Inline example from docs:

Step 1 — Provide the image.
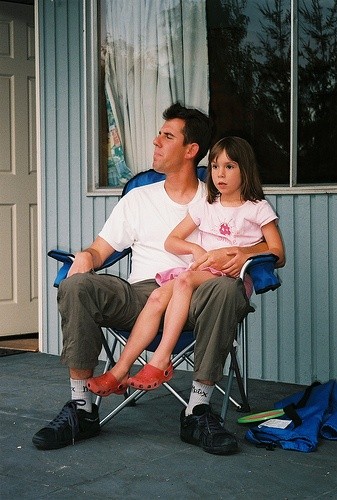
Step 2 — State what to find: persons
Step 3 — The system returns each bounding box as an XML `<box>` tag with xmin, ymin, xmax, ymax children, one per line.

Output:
<box><xmin>86</xmin><ymin>137</ymin><xmax>285</xmax><ymax>396</ymax></box>
<box><xmin>31</xmin><ymin>99</ymin><xmax>268</xmax><ymax>456</ymax></box>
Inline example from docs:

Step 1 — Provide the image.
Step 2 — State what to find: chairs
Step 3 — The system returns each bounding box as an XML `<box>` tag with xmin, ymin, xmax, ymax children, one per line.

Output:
<box><xmin>46</xmin><ymin>167</ymin><xmax>283</xmax><ymax>429</ymax></box>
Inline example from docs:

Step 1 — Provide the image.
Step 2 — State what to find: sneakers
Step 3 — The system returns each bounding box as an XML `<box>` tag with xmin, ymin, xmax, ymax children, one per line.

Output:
<box><xmin>180</xmin><ymin>403</ymin><xmax>238</xmax><ymax>453</ymax></box>
<box><xmin>31</xmin><ymin>399</ymin><xmax>101</xmax><ymax>449</ymax></box>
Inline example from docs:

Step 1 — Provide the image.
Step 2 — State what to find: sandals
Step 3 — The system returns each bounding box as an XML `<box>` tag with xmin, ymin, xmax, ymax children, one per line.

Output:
<box><xmin>87</xmin><ymin>370</ymin><xmax>126</xmax><ymax>397</ymax></box>
<box><xmin>127</xmin><ymin>361</ymin><xmax>175</xmax><ymax>390</ymax></box>
